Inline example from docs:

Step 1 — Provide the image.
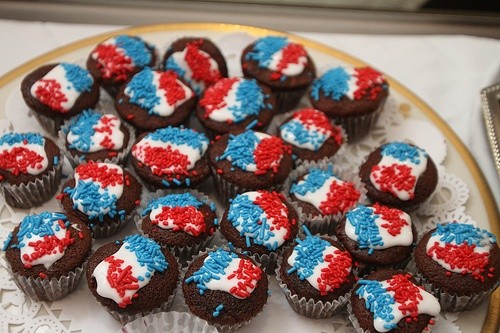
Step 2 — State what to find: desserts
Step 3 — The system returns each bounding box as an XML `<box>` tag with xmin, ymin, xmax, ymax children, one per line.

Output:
<box><xmin>0</xmin><ymin>34</ymin><xmax>500</xmax><ymax>333</ymax></box>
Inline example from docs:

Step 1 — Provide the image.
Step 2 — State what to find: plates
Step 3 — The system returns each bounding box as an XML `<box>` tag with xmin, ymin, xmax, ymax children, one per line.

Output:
<box><xmin>0</xmin><ymin>23</ymin><xmax>500</xmax><ymax>333</ymax></box>
